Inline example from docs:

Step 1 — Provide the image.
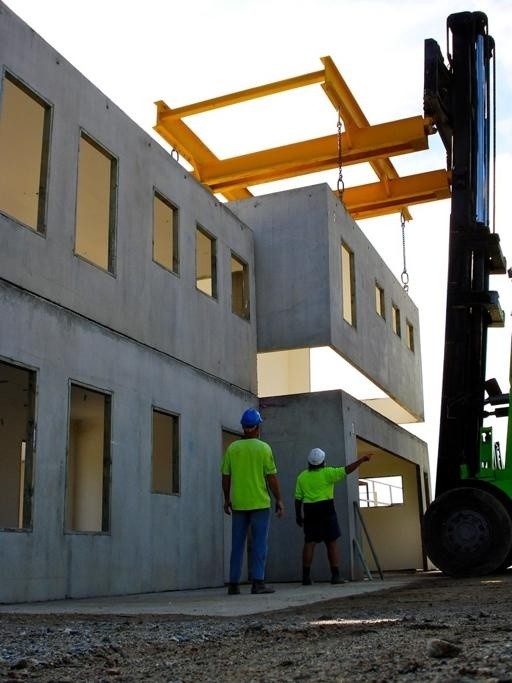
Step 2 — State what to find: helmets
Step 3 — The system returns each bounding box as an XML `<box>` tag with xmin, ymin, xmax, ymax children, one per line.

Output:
<box><xmin>306</xmin><ymin>447</ymin><xmax>326</xmax><ymax>466</ymax></box>
<box><xmin>240</xmin><ymin>407</ymin><xmax>263</xmax><ymax>425</ymax></box>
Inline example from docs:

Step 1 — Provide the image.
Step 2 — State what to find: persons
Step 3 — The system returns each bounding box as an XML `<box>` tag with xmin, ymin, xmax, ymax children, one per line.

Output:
<box><xmin>222</xmin><ymin>408</ymin><xmax>284</xmax><ymax>594</ymax></box>
<box><xmin>294</xmin><ymin>448</ymin><xmax>372</xmax><ymax>585</ymax></box>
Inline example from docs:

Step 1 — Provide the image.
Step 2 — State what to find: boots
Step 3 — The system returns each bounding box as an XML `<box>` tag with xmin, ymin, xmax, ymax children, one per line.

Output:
<box><xmin>302</xmin><ymin>565</ymin><xmax>312</xmax><ymax>585</ymax></box>
<box><xmin>331</xmin><ymin>566</ymin><xmax>350</xmax><ymax>583</ymax></box>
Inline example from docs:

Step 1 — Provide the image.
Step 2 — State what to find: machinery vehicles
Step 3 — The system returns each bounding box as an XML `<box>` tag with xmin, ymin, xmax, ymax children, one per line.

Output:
<box><xmin>152</xmin><ymin>10</ymin><xmax>511</xmax><ymax>586</ymax></box>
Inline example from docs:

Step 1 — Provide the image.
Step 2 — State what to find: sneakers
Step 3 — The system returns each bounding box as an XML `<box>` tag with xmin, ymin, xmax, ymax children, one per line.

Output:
<box><xmin>251</xmin><ymin>582</ymin><xmax>275</xmax><ymax>594</ymax></box>
<box><xmin>228</xmin><ymin>583</ymin><xmax>241</xmax><ymax>595</ymax></box>
